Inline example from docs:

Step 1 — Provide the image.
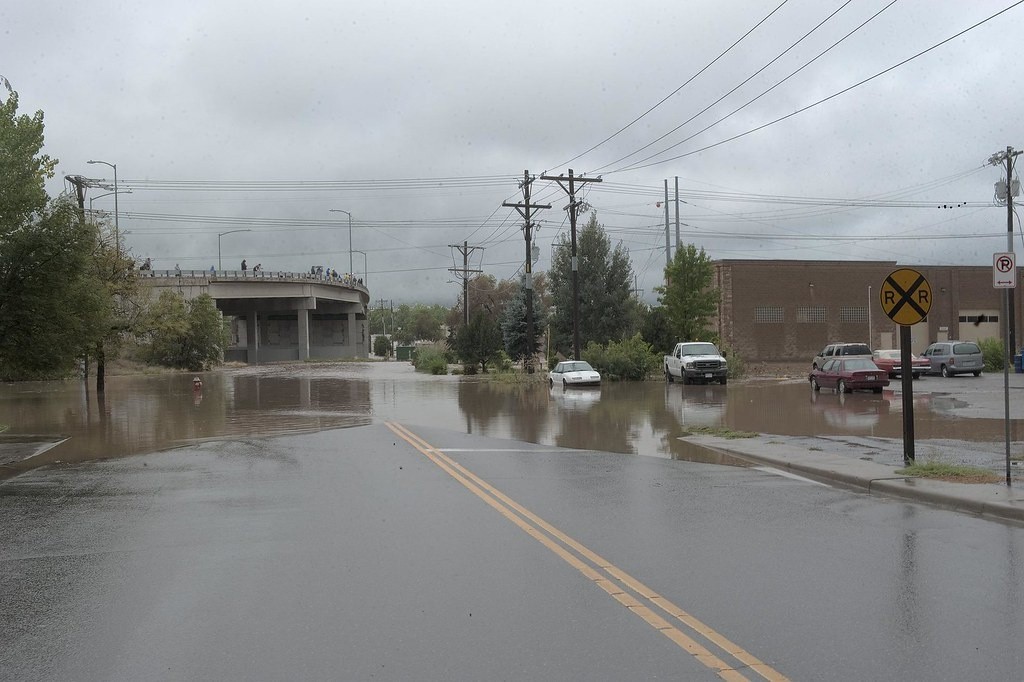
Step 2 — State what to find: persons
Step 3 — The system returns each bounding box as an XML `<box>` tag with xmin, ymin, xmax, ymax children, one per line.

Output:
<box><xmin>140</xmin><ymin>257</ymin><xmax>151</xmax><ymax>270</ymax></box>
<box><xmin>241</xmin><ymin>259</ymin><xmax>247</xmax><ymax>276</ymax></box>
<box><xmin>174</xmin><ymin>264</ymin><xmax>180</xmax><ymax>277</ymax></box>
<box><xmin>210</xmin><ymin>266</ymin><xmax>216</xmax><ymax>278</ymax></box>
<box><xmin>311</xmin><ymin>266</ymin><xmax>363</xmax><ymax>285</ymax></box>
<box><xmin>253</xmin><ymin>264</ymin><xmax>261</xmax><ymax>276</ymax></box>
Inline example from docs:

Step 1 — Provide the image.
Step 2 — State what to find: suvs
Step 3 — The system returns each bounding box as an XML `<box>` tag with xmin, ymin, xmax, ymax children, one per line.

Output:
<box><xmin>817</xmin><ymin>343</ymin><xmax>873</xmax><ymax>360</ymax></box>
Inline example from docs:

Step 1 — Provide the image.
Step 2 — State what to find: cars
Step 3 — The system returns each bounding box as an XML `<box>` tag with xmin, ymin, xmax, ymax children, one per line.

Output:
<box><xmin>547</xmin><ymin>360</ymin><xmax>601</xmax><ymax>387</ymax></box>
<box><xmin>808</xmin><ymin>356</ymin><xmax>890</xmax><ymax>394</ymax></box>
<box><xmin>872</xmin><ymin>349</ymin><xmax>932</xmax><ymax>379</ymax></box>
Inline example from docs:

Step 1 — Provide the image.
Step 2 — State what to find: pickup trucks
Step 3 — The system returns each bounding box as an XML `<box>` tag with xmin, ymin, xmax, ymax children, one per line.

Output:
<box><xmin>663</xmin><ymin>342</ymin><xmax>729</xmax><ymax>385</ymax></box>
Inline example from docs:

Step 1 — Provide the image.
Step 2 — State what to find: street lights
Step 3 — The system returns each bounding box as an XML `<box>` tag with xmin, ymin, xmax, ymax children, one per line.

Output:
<box><xmin>87</xmin><ymin>160</ymin><xmax>119</xmax><ymax>258</ymax></box>
<box><xmin>329</xmin><ymin>209</ymin><xmax>352</xmax><ymax>282</ymax></box>
<box><xmin>89</xmin><ymin>191</ymin><xmax>136</xmax><ymax>226</ymax></box>
<box><xmin>351</xmin><ymin>250</ymin><xmax>367</xmax><ymax>288</ymax></box>
<box><xmin>219</xmin><ymin>229</ymin><xmax>251</xmax><ymax>277</ymax></box>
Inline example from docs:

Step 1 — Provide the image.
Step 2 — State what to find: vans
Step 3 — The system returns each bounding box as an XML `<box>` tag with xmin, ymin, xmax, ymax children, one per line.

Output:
<box><xmin>920</xmin><ymin>341</ymin><xmax>985</xmax><ymax>378</ymax></box>
